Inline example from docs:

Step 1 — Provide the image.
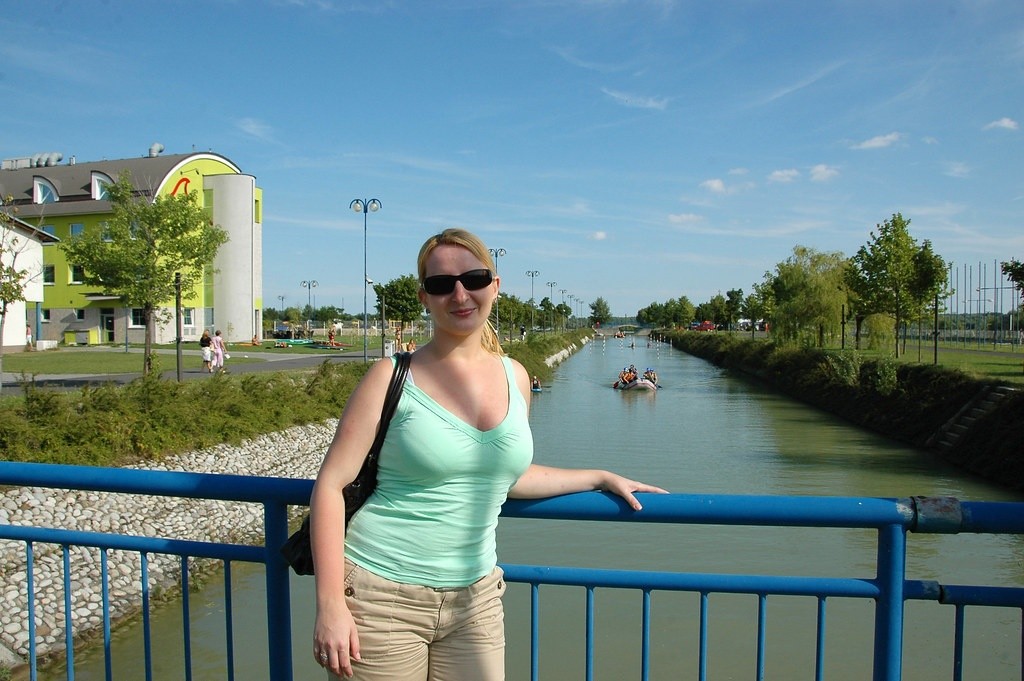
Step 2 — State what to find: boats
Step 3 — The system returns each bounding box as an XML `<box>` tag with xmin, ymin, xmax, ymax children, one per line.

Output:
<box><xmin>622</xmin><ymin>376</ymin><xmax>657</xmax><ymax>391</ymax></box>
<box><xmin>593</xmin><ymin>333</ymin><xmax>604</xmax><ymax>337</ymax></box>
<box><xmin>531</xmin><ymin>386</ymin><xmax>542</xmax><ymax>393</ymax></box>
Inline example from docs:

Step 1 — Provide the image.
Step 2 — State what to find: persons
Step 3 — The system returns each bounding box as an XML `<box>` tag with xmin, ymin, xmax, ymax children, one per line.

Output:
<box><xmin>198</xmin><ymin>330</ymin><xmax>213</xmax><ymax>373</ymax></box>
<box><xmin>310</xmin><ymin>228</ymin><xmax>671</xmax><ymax>681</ymax></box>
<box><xmin>26</xmin><ymin>324</ymin><xmax>33</xmax><ymax>352</ymax></box>
<box><xmin>209</xmin><ymin>330</ymin><xmax>228</xmax><ymax>374</ymax></box>
<box><xmin>252</xmin><ymin>335</ymin><xmax>262</xmax><ymax>346</ymax></box>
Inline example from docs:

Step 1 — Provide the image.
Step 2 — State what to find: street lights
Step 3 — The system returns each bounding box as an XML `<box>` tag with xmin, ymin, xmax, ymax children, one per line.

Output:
<box><xmin>300</xmin><ymin>279</ymin><xmax>319</xmax><ymax>320</ymax></box>
<box><xmin>525</xmin><ymin>270</ymin><xmax>540</xmax><ymax>328</ymax></box>
<box><xmin>545</xmin><ymin>281</ymin><xmax>557</xmax><ymax>332</ymax></box>
<box><xmin>278</xmin><ymin>295</ymin><xmax>286</xmax><ymax>312</ymax></box>
<box><xmin>568</xmin><ymin>295</ymin><xmax>585</xmax><ymax>329</ymax></box>
<box><xmin>962</xmin><ymin>298</ymin><xmax>993</xmax><ymax>343</ymax></box>
<box><xmin>348</xmin><ymin>197</ymin><xmax>382</xmax><ymax>363</ymax></box>
<box><xmin>487</xmin><ymin>248</ymin><xmax>507</xmax><ymax>329</ymax></box>
<box><xmin>559</xmin><ymin>289</ymin><xmax>567</xmax><ymax>333</ymax></box>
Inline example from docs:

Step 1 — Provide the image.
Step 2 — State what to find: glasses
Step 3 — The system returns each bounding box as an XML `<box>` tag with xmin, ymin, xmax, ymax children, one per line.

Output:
<box><xmin>424</xmin><ymin>268</ymin><xmax>494</xmax><ymax>295</ymax></box>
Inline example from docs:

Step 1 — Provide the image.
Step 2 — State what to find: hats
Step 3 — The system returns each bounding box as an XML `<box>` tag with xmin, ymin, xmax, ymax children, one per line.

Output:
<box><xmin>650</xmin><ymin>369</ymin><xmax>653</xmax><ymax>372</ymax></box>
<box><xmin>624</xmin><ymin>367</ymin><xmax>628</xmax><ymax>371</ymax></box>
<box><xmin>647</xmin><ymin>368</ymin><xmax>650</xmax><ymax>371</ymax></box>
<box><xmin>630</xmin><ymin>364</ymin><xmax>634</xmax><ymax>368</ymax></box>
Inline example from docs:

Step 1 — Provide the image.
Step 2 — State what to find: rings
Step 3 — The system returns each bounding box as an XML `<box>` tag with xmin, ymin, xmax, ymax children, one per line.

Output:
<box><xmin>319</xmin><ymin>653</ymin><xmax>328</xmax><ymax>659</ymax></box>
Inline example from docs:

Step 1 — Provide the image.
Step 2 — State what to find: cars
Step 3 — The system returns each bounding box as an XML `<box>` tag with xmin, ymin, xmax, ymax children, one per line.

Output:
<box><xmin>690</xmin><ymin>320</ymin><xmax>767</xmax><ymax>331</ymax></box>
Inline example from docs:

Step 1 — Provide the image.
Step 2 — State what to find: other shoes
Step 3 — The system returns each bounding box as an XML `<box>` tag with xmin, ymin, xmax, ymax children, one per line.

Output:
<box><xmin>209</xmin><ymin>367</ymin><xmax>212</xmax><ymax>373</ymax></box>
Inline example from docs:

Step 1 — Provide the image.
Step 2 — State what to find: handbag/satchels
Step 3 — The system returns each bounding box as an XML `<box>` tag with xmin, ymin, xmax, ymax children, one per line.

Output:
<box><xmin>282</xmin><ymin>350</ymin><xmax>412</xmax><ymax>575</ymax></box>
